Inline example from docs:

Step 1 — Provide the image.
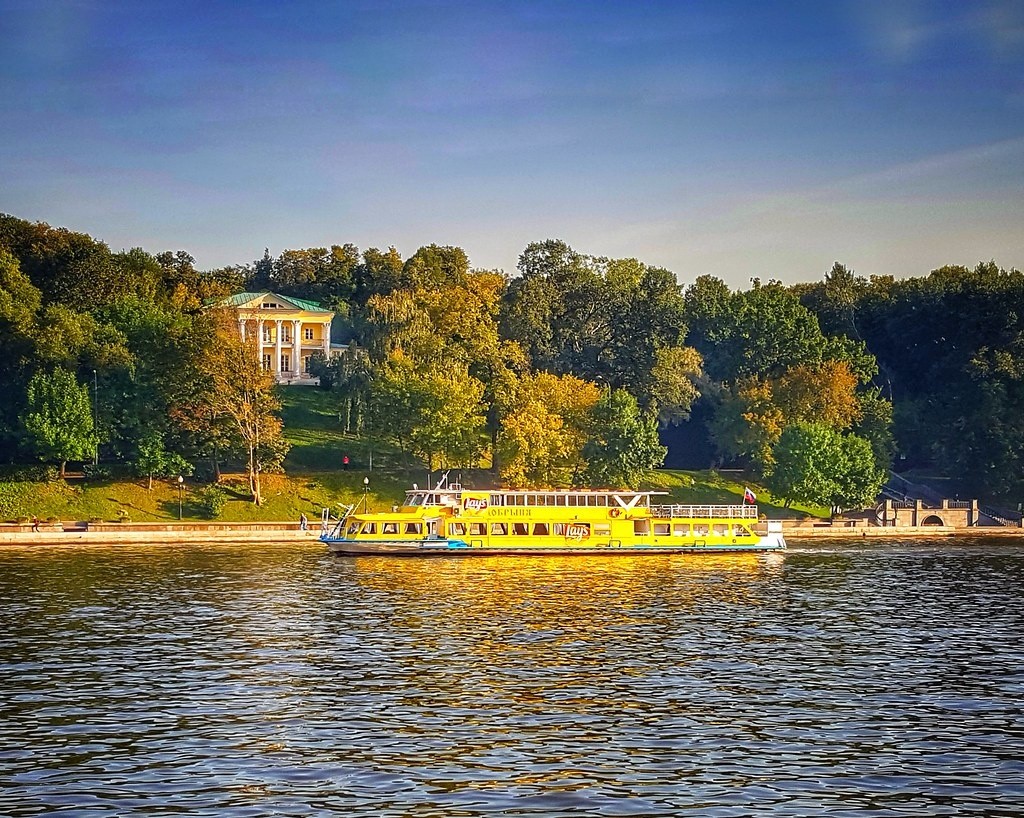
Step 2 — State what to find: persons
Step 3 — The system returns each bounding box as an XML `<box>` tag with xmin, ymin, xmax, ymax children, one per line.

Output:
<box><xmin>343</xmin><ymin>455</ymin><xmax>349</xmax><ymax>470</ymax></box>
<box><xmin>691</xmin><ymin>478</ymin><xmax>694</xmax><ymax>487</ymax></box>
<box><xmin>300</xmin><ymin>513</ymin><xmax>308</xmax><ymax>530</ymax></box>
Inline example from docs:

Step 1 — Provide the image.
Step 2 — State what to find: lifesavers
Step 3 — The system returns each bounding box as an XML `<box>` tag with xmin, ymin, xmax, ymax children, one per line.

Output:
<box><xmin>611</xmin><ymin>508</ymin><xmax>620</xmax><ymax>518</ymax></box>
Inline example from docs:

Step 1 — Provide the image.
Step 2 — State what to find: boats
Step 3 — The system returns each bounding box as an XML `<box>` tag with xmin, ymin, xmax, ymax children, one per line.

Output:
<box><xmin>320</xmin><ymin>474</ymin><xmax>786</xmax><ymax>557</ymax></box>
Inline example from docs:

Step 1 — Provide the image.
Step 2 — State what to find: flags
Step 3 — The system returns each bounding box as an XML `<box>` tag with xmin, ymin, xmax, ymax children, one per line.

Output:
<box><xmin>745</xmin><ymin>488</ymin><xmax>756</xmax><ymax>504</ymax></box>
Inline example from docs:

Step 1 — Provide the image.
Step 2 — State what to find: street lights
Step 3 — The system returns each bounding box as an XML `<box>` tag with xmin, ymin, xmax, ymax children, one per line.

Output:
<box><xmin>363</xmin><ymin>476</ymin><xmax>369</xmax><ymax>514</ymax></box>
<box><xmin>691</xmin><ymin>478</ymin><xmax>694</xmax><ymax>502</ymax></box>
<box><xmin>90</xmin><ymin>369</ymin><xmax>102</xmax><ymax>465</ymax></box>
<box><xmin>175</xmin><ymin>475</ymin><xmax>187</xmax><ymax>520</ymax></box>
